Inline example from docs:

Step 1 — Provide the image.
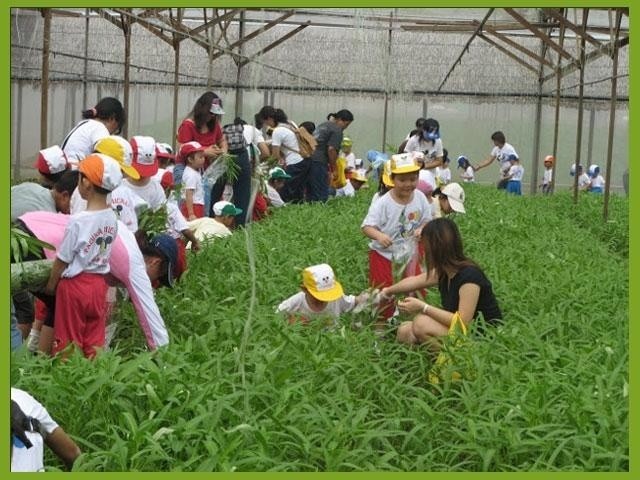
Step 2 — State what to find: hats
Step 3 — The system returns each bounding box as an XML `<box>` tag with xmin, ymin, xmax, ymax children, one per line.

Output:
<box><xmin>213</xmin><ymin>200</ymin><xmax>244</xmax><ymax>216</ymax></box>
<box><xmin>351</xmin><ymin>150</ymin><xmax>388</xmax><ymax>183</ymax></box>
<box><xmin>302</xmin><ymin>264</ymin><xmax>343</xmax><ymax>302</ymax></box>
<box><xmin>268</xmin><ymin>167</ymin><xmax>291</xmax><ymax>179</ymax></box>
<box><xmin>441</xmin><ymin>183</ymin><xmax>466</xmax><ymax>214</ymax></box>
<box><xmin>543</xmin><ymin>155</ymin><xmax>553</xmax><ymax>162</ymax></box>
<box><xmin>457</xmin><ymin>156</ymin><xmax>468</xmax><ymax>169</ymax></box>
<box><xmin>150</xmin><ymin>233</ymin><xmax>176</xmax><ymax>289</ymax></box>
<box><xmin>31</xmin><ymin>145</ymin><xmax>68</xmax><ymax>174</ymax></box>
<box><xmin>423</xmin><ymin>119</ymin><xmax>439</xmax><ymax>140</ymax></box>
<box><xmin>382</xmin><ymin>151</ymin><xmax>426</xmax><ymax>187</ymax></box>
<box><xmin>208</xmin><ymin>98</ymin><xmax>225</xmax><ymax>115</ymax></box>
<box><xmin>569</xmin><ymin>164</ymin><xmax>599</xmax><ymax>176</ymax></box>
<box><xmin>505</xmin><ymin>154</ymin><xmax>520</xmax><ymax>160</ymax></box>
<box><xmin>66</xmin><ymin>135</ymin><xmax>209</xmax><ymax>188</ymax></box>
<box><xmin>340</xmin><ymin>137</ymin><xmax>352</xmax><ymax>148</ymax></box>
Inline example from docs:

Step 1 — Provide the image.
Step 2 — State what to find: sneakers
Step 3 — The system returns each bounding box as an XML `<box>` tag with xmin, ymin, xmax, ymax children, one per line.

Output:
<box><xmin>26</xmin><ymin>329</ymin><xmax>40</xmax><ymax>352</ymax></box>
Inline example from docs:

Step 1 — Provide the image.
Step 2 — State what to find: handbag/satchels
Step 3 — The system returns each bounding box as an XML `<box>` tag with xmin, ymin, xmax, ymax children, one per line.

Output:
<box><xmin>428</xmin><ymin>312</ymin><xmax>468</xmax><ymax>389</ymax></box>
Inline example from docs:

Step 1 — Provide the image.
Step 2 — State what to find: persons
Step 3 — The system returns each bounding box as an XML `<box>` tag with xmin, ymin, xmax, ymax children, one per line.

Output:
<box><xmin>569</xmin><ymin>163</ymin><xmax>591</xmax><ymax>193</ymax></box>
<box><xmin>472</xmin><ymin>131</ymin><xmax>520</xmax><ymax>190</ymax></box>
<box><xmin>587</xmin><ymin>164</ymin><xmax>606</xmax><ymax>194</ymax></box>
<box><xmin>374</xmin><ymin>215</ymin><xmax>508</xmax><ymax>354</ymax></box>
<box><xmin>361</xmin><ymin>117</ymin><xmax>468</xmax><ymax>320</ymax></box>
<box><xmin>9</xmin><ymin>89</ymin><xmax>370</xmax><ymax>361</ymax></box>
<box><xmin>538</xmin><ymin>155</ymin><xmax>556</xmax><ymax>195</ymax></box>
<box><xmin>500</xmin><ymin>153</ymin><xmax>526</xmax><ymax>196</ymax></box>
<box><xmin>456</xmin><ymin>155</ymin><xmax>475</xmax><ymax>184</ymax></box>
<box><xmin>10</xmin><ymin>385</ymin><xmax>84</xmax><ymax>473</ymax></box>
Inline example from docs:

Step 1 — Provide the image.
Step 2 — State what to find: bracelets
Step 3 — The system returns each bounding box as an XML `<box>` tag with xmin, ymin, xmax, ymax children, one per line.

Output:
<box><xmin>422</xmin><ymin>303</ymin><xmax>430</xmax><ymax>314</ymax></box>
<box><xmin>381</xmin><ymin>287</ymin><xmax>395</xmax><ymax>300</ymax></box>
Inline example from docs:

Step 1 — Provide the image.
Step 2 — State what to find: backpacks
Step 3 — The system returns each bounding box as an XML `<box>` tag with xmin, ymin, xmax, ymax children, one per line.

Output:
<box><xmin>223</xmin><ymin>124</ymin><xmax>246</xmax><ymax>154</ymax></box>
<box><xmin>295</xmin><ymin>127</ymin><xmax>317</xmax><ymax>158</ymax></box>
